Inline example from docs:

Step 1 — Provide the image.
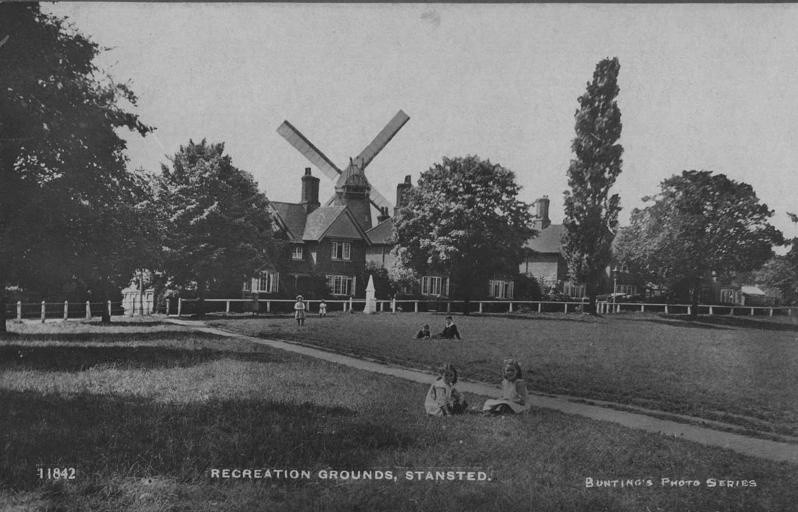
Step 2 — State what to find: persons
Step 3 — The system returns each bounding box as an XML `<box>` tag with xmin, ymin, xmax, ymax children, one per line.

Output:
<box><xmin>250</xmin><ymin>292</ymin><xmax>260</xmax><ymax>316</ymax></box>
<box><xmin>414</xmin><ymin>323</ymin><xmax>429</xmax><ymax>340</ymax></box>
<box><xmin>482</xmin><ymin>358</ymin><xmax>530</xmax><ymax>416</ymax></box>
<box><xmin>430</xmin><ymin>315</ymin><xmax>463</xmax><ymax>340</ymax></box>
<box><xmin>319</xmin><ymin>298</ymin><xmax>327</xmax><ymax>317</ymax></box>
<box><xmin>423</xmin><ymin>363</ymin><xmax>468</xmax><ymax>417</ymax></box>
<box><xmin>293</xmin><ymin>294</ymin><xmax>306</xmax><ymax>326</ymax></box>
